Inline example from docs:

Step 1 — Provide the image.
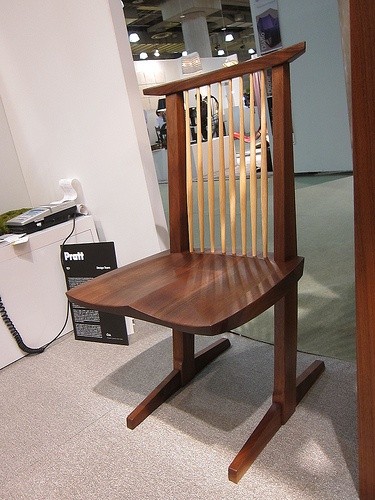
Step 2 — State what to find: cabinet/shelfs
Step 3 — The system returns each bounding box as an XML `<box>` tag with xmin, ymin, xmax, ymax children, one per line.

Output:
<box><xmin>0</xmin><ymin>215</ymin><xmax>101</xmax><ymax>369</ymax></box>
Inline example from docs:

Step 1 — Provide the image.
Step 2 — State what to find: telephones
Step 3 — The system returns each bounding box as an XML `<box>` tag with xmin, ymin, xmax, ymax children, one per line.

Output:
<box><xmin>5</xmin><ymin>200</ymin><xmax>77</xmax><ymax>234</ymax></box>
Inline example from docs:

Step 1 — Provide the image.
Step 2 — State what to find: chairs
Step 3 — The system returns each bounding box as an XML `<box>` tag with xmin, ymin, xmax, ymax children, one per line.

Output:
<box><xmin>65</xmin><ymin>41</ymin><xmax>326</xmax><ymax>484</ymax></box>
